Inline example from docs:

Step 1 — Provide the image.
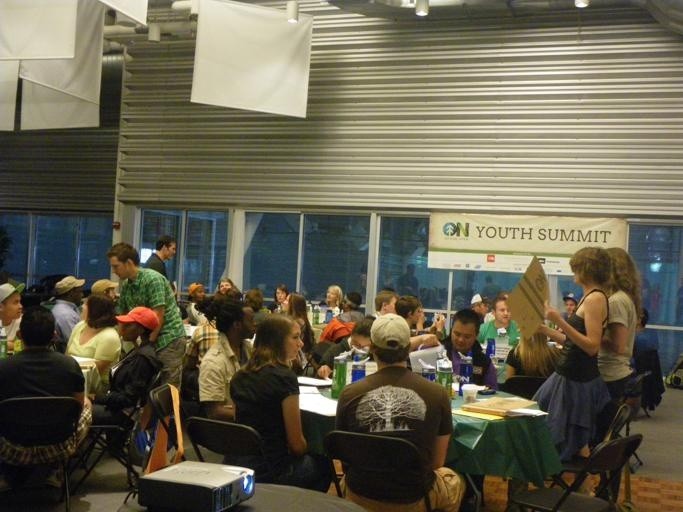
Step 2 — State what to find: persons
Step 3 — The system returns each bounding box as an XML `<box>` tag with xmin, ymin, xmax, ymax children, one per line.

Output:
<box><xmin>3</xmin><ymin>276</ymin><xmax>162</xmax><ymax>512</ymax></box>
<box><xmin>335</xmin><ymin>313</ymin><xmax>466</xmax><ymax>510</ymax></box>
<box><xmin>478</xmin><ymin>290</ymin><xmax>518</xmax><ymax>347</ymax></box>
<box><xmin>587</xmin><ymin>246</ymin><xmax>645</xmax><ymax>496</ymax></box>
<box><xmin>142</xmin><ymin>234</ymin><xmax>177</xmax><ymax>280</ymax></box>
<box><xmin>223</xmin><ymin>313</ymin><xmax>333</xmax><ymax>494</ymax></box>
<box><xmin>505</xmin><ymin>321</ymin><xmax>564</xmax><ymax>379</ymax></box>
<box><xmin>531</xmin><ymin>246</ymin><xmax>612</xmax><ymax>497</ymax></box>
<box><xmin>108</xmin><ymin>243</ymin><xmax>187</xmax><ymax>450</ymax></box>
<box><xmin>633</xmin><ymin>307</ymin><xmax>660</xmax><ymax>352</ymax></box>
<box><xmin>560</xmin><ymin>292</ymin><xmax>579</xmax><ymax>321</ymax></box>
<box><xmin>183</xmin><ymin>276</ymin><xmax>497</xmax><ymax>422</ymax></box>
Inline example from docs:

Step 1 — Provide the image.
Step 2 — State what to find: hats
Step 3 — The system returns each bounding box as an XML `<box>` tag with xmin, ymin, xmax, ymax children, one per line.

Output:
<box><xmin>187</xmin><ymin>283</ymin><xmax>203</xmax><ymax>301</ymax></box>
<box><xmin>344</xmin><ymin>292</ymin><xmax>362</xmax><ymax>309</ymax></box>
<box><xmin>371</xmin><ymin>314</ymin><xmax>410</xmax><ymax>349</ymax></box>
<box><xmin>92</xmin><ymin>280</ymin><xmax>118</xmax><ymax>298</ymax></box>
<box><xmin>0</xmin><ymin>283</ymin><xmax>25</xmax><ymax>304</ymax></box>
<box><xmin>116</xmin><ymin>307</ymin><xmax>159</xmax><ymax>331</ymax></box>
<box><xmin>55</xmin><ymin>275</ymin><xmax>86</xmax><ymax>295</ymax></box>
<box><xmin>470</xmin><ymin>293</ymin><xmax>489</xmax><ymax>306</ymax></box>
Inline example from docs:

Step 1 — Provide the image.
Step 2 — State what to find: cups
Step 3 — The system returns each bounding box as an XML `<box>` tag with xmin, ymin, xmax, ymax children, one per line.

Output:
<box><xmin>461</xmin><ymin>384</ymin><xmax>477</xmax><ymax>402</ymax></box>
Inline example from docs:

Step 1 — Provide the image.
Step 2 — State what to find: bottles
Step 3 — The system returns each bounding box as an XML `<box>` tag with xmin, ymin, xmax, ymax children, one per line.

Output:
<box><xmin>436</xmin><ymin>361</ymin><xmax>453</xmax><ymax>399</ymax></box>
<box><xmin>331</xmin><ymin>355</ymin><xmax>347</xmax><ymax>397</ymax></box>
<box><xmin>458</xmin><ymin>352</ymin><xmax>473</xmax><ymax>396</ymax></box>
<box><xmin>486</xmin><ymin>338</ymin><xmax>495</xmax><ymax>359</ymax></box>
<box><xmin>312</xmin><ymin>305</ymin><xmax>319</xmax><ymax>325</ymax></box>
<box><xmin>325</xmin><ymin>310</ymin><xmax>332</xmax><ymax>323</ymax></box>
<box><xmin>0</xmin><ymin>333</ymin><xmax>7</xmax><ymax>361</ymax></box>
<box><xmin>351</xmin><ymin>361</ymin><xmax>365</xmax><ymax>381</ymax></box>
<box><xmin>421</xmin><ymin>367</ymin><xmax>435</xmax><ymax>383</ymax></box>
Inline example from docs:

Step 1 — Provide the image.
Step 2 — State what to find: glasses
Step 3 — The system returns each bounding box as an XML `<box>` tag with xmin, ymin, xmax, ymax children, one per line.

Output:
<box><xmin>562</xmin><ymin>292</ymin><xmax>577</xmax><ymax>305</ymax></box>
<box><xmin>350</xmin><ymin>342</ymin><xmax>370</xmax><ymax>349</ymax></box>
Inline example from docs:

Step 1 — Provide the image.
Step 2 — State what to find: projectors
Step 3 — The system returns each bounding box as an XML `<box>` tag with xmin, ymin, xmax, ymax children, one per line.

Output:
<box><xmin>138</xmin><ymin>461</ymin><xmax>255</xmax><ymax>512</ymax></box>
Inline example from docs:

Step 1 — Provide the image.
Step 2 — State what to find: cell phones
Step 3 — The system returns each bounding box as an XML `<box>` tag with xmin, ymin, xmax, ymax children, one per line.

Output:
<box><xmin>478</xmin><ymin>388</ymin><xmax>496</xmax><ymax>395</ymax></box>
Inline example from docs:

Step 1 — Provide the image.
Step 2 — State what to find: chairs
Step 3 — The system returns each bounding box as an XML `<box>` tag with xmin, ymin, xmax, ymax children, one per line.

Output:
<box><xmin>0</xmin><ymin>324</ymin><xmax>653</xmax><ymax>512</ymax></box>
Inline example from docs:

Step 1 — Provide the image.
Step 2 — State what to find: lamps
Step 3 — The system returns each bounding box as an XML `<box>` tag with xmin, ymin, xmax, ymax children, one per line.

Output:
<box><xmin>146</xmin><ymin>21</ymin><xmax>161</xmax><ymax>44</ymax></box>
<box><xmin>168</xmin><ymin>1</ymin><xmax>592</xmax><ymax>26</ymax></box>
